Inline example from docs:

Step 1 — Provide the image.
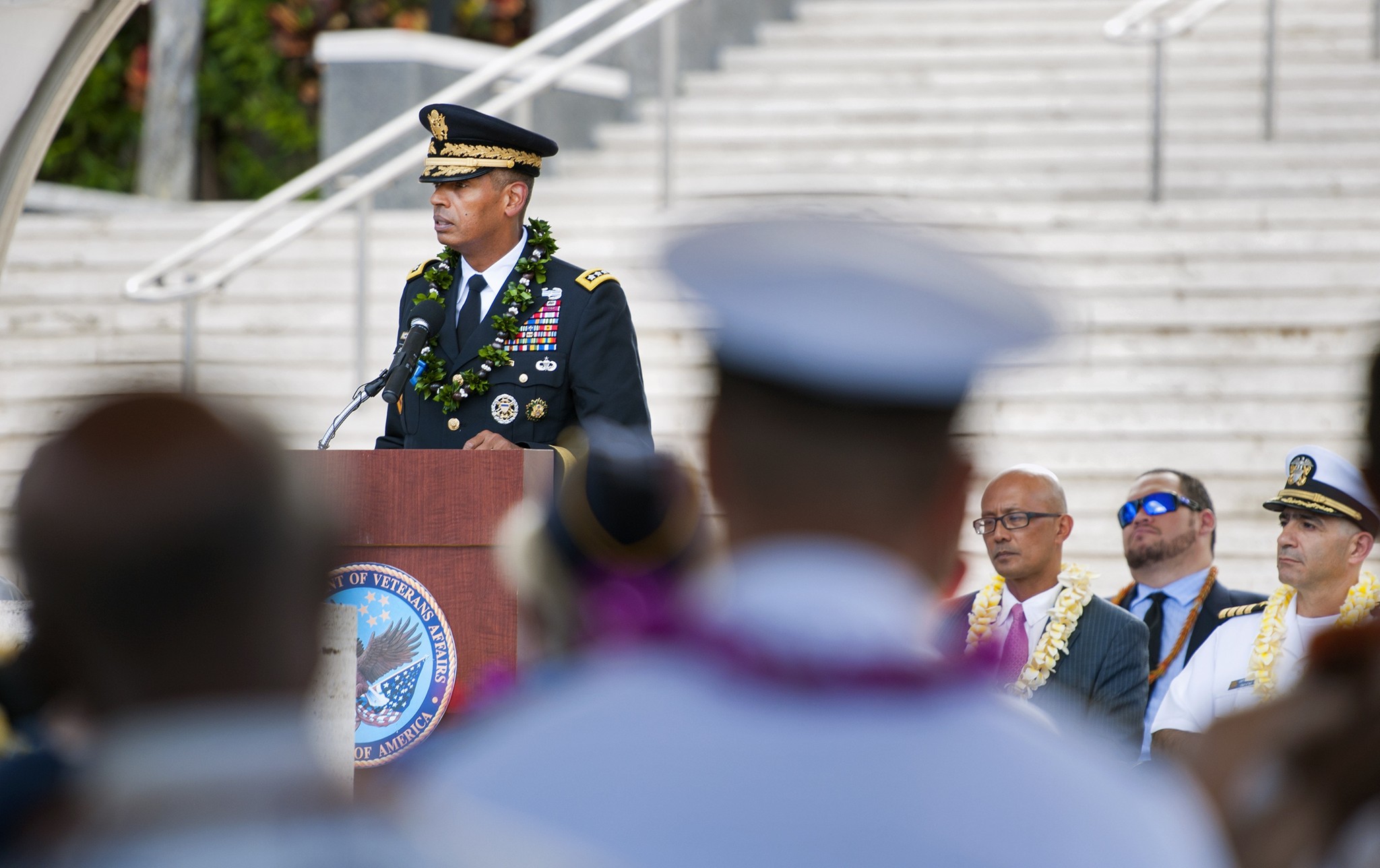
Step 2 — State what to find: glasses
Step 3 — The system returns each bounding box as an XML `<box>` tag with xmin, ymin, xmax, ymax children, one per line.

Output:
<box><xmin>972</xmin><ymin>511</ymin><xmax>1066</xmax><ymax>534</ymax></box>
<box><xmin>1117</xmin><ymin>493</ymin><xmax>1204</xmax><ymax>528</ymax></box>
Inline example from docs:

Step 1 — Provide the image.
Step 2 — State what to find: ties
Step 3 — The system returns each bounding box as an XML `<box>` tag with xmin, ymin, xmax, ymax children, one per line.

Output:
<box><xmin>1142</xmin><ymin>589</ymin><xmax>1169</xmax><ymax>714</ymax></box>
<box><xmin>456</xmin><ymin>273</ymin><xmax>488</xmax><ymax>350</ymax></box>
<box><xmin>999</xmin><ymin>603</ymin><xmax>1029</xmax><ymax>686</ymax></box>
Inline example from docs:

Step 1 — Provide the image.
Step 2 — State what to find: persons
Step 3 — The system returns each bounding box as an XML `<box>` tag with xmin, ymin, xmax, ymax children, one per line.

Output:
<box><xmin>0</xmin><ymin>387</ymin><xmax>404</xmax><ymax>868</ymax></box>
<box><xmin>375</xmin><ymin>103</ymin><xmax>653</xmax><ymax>493</ymax></box>
<box><xmin>1102</xmin><ymin>469</ymin><xmax>1267</xmax><ymax>764</ymax></box>
<box><xmin>1151</xmin><ymin>444</ymin><xmax>1380</xmax><ymax>786</ymax></box>
<box><xmin>947</xmin><ymin>465</ymin><xmax>1148</xmax><ymax>766</ymax></box>
<box><xmin>489</xmin><ymin>449</ymin><xmax>710</xmax><ymax>643</ymax></box>
<box><xmin>403</xmin><ymin>218</ymin><xmax>1230</xmax><ymax>867</ymax></box>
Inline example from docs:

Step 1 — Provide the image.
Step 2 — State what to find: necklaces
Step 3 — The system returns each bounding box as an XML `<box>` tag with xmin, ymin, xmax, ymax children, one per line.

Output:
<box><xmin>966</xmin><ymin>562</ymin><xmax>1092</xmax><ymax>701</ymax></box>
<box><xmin>573</xmin><ymin>575</ymin><xmax>1026</xmax><ymax>705</ymax></box>
<box><xmin>412</xmin><ymin>218</ymin><xmax>557</xmax><ymax>414</ymax></box>
<box><xmin>1249</xmin><ymin>571</ymin><xmax>1380</xmax><ymax>704</ymax></box>
<box><xmin>1112</xmin><ymin>566</ymin><xmax>1217</xmax><ymax>681</ymax></box>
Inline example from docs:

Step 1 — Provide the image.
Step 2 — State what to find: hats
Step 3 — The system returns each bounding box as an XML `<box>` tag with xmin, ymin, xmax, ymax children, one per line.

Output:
<box><xmin>1262</xmin><ymin>446</ymin><xmax>1380</xmax><ymax>538</ymax></box>
<box><xmin>490</xmin><ymin>417</ymin><xmax>725</xmax><ymax>638</ymax></box>
<box><xmin>669</xmin><ymin>225</ymin><xmax>1051</xmax><ymax>422</ymax></box>
<box><xmin>417</xmin><ymin>103</ymin><xmax>560</xmax><ymax>181</ymax></box>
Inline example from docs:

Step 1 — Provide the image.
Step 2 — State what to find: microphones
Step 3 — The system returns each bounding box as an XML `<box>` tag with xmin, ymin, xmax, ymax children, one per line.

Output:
<box><xmin>383</xmin><ymin>300</ymin><xmax>442</xmax><ymax>404</ymax></box>
